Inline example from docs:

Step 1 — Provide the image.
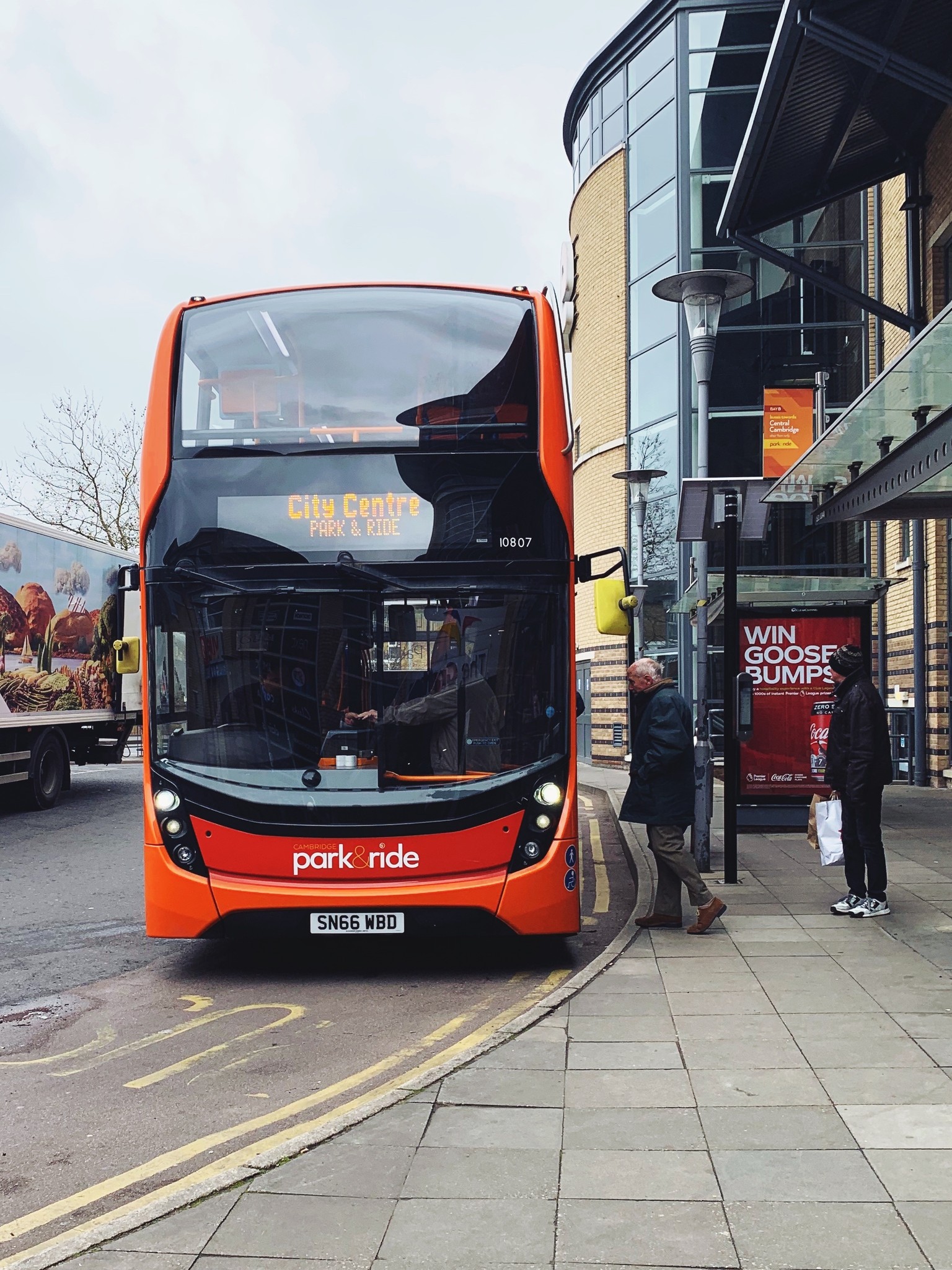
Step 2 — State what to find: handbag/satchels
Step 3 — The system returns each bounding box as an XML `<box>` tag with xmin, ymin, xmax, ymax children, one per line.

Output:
<box><xmin>807</xmin><ymin>791</ymin><xmax>836</xmax><ymax>849</ymax></box>
<box><xmin>814</xmin><ymin>790</ymin><xmax>845</xmax><ymax>866</ymax></box>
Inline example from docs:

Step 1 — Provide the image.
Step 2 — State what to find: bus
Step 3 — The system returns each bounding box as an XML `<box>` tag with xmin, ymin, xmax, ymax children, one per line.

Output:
<box><xmin>113</xmin><ymin>279</ymin><xmax>639</xmax><ymax>939</ymax></box>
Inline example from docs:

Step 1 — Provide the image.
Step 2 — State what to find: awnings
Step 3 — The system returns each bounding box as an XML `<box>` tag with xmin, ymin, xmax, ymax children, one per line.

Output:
<box><xmin>714</xmin><ymin>0</ymin><xmax>952</xmax><ymax>332</ymax></box>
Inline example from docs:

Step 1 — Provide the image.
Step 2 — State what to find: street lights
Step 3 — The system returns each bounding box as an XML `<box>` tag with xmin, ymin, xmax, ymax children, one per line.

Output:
<box><xmin>613</xmin><ymin>468</ymin><xmax>668</xmax><ymax>664</ymax></box>
<box><xmin>652</xmin><ymin>268</ymin><xmax>760</xmax><ymax>874</ymax></box>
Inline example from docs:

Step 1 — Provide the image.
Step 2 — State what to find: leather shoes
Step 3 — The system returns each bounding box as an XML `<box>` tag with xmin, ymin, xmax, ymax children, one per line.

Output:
<box><xmin>634</xmin><ymin>914</ymin><xmax>682</xmax><ymax>928</ymax></box>
<box><xmin>687</xmin><ymin>897</ymin><xmax>727</xmax><ymax>935</ymax></box>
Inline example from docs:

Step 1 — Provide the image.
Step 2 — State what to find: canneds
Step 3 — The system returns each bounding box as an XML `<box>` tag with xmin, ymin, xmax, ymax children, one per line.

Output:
<box><xmin>810</xmin><ymin>701</ymin><xmax>835</xmax><ymax>781</ymax></box>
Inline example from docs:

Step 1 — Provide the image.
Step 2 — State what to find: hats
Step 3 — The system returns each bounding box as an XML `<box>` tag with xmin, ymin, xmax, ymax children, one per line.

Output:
<box><xmin>829</xmin><ymin>643</ymin><xmax>862</xmax><ymax>676</ymax></box>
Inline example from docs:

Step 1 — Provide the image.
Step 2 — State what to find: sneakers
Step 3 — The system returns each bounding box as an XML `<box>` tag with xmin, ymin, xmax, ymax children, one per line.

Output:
<box><xmin>830</xmin><ymin>894</ymin><xmax>866</xmax><ymax>914</ymax></box>
<box><xmin>849</xmin><ymin>899</ymin><xmax>891</xmax><ymax>918</ymax></box>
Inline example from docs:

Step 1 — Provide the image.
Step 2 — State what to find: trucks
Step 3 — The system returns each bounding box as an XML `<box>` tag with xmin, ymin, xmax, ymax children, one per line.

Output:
<box><xmin>0</xmin><ymin>512</ymin><xmax>144</xmax><ymax>811</ymax></box>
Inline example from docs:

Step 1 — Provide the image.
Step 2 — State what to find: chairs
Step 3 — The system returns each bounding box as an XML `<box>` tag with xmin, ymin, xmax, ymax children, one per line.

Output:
<box><xmin>424</xmin><ymin>402</ymin><xmax>530</xmax><ymax>442</ymax></box>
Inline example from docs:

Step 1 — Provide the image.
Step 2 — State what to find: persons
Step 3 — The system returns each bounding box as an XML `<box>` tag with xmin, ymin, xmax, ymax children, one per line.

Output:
<box><xmin>218</xmin><ymin>654</ymin><xmax>357</xmax><ymax>768</ymax></box>
<box><xmin>357</xmin><ymin>647</ymin><xmax>503</xmax><ymax>785</ymax></box>
<box><xmin>576</xmin><ymin>691</ymin><xmax>585</xmax><ymax>718</ymax></box>
<box><xmin>824</xmin><ymin>643</ymin><xmax>891</xmax><ymax>917</ymax></box>
<box><xmin>616</xmin><ymin>658</ymin><xmax>728</xmax><ymax>934</ymax></box>
